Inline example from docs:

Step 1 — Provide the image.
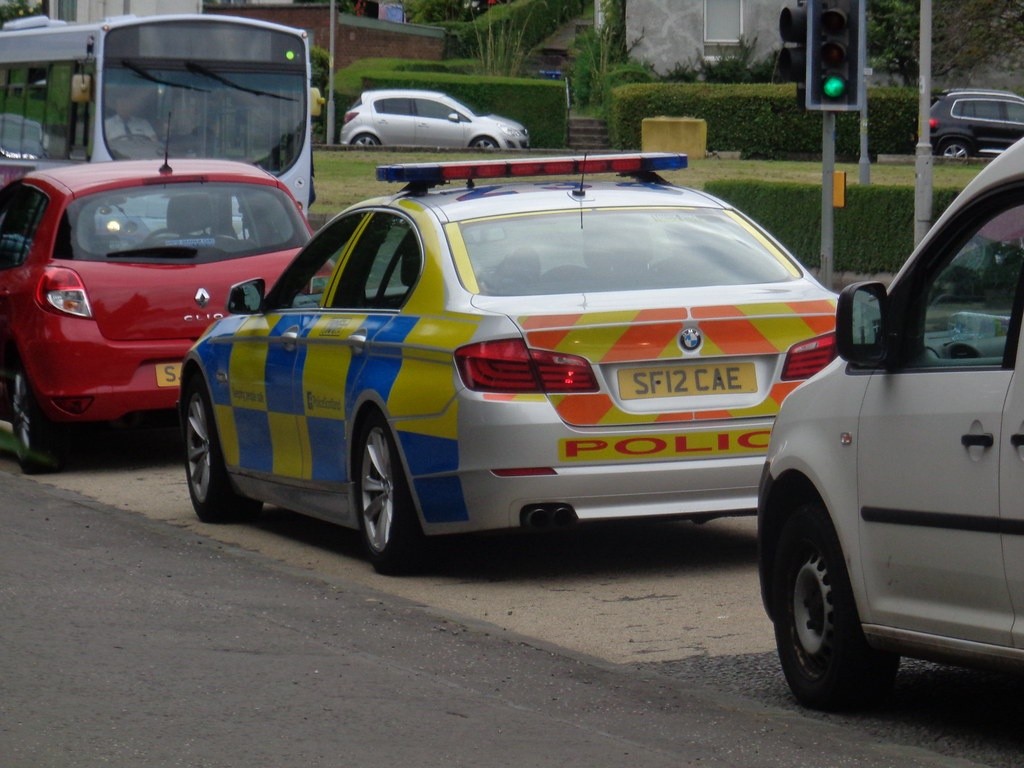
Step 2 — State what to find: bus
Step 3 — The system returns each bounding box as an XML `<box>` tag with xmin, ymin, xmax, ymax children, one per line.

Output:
<box><xmin>0</xmin><ymin>14</ymin><xmax>324</xmax><ymax>220</ymax></box>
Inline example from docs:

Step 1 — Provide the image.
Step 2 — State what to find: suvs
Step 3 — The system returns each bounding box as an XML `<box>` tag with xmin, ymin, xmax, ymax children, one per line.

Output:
<box><xmin>913</xmin><ymin>87</ymin><xmax>1024</xmax><ymax>170</ymax></box>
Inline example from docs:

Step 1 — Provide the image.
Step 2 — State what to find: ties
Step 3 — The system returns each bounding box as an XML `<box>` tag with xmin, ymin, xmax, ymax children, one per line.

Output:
<box><xmin>121</xmin><ymin>120</ymin><xmax>131</xmax><ymax>137</ymax></box>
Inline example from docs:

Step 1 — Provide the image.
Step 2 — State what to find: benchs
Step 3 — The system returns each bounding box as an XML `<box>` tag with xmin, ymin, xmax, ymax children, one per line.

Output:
<box><xmin>481</xmin><ymin>242</ymin><xmax>764</xmax><ymax>301</ymax></box>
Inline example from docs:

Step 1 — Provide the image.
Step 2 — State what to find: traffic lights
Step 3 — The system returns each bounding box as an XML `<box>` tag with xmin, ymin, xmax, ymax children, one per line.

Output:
<box><xmin>806</xmin><ymin>0</ymin><xmax>867</xmax><ymax>111</ymax></box>
<box><xmin>779</xmin><ymin>0</ymin><xmax>807</xmax><ymax>109</ymax></box>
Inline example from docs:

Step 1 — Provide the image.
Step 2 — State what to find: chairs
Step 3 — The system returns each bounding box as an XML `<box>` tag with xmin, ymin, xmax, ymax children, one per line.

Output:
<box><xmin>558</xmin><ymin>220</ymin><xmax>655</xmax><ymax>295</ymax></box>
<box><xmin>151</xmin><ymin>191</ymin><xmax>235</xmax><ymax>244</ymax></box>
<box><xmin>367</xmin><ymin>228</ymin><xmax>498</xmax><ymax>307</ymax></box>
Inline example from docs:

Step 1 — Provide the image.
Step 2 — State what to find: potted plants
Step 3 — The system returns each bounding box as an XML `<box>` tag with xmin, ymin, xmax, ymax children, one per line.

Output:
<box><xmin>566</xmin><ymin>26</ymin><xmax>648</xmax><ymax>150</ymax></box>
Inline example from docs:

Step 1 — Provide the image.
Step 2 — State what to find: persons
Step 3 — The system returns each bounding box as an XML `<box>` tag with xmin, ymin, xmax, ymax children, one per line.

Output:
<box><xmin>104</xmin><ymin>90</ymin><xmax>157</xmax><ymax>142</ymax></box>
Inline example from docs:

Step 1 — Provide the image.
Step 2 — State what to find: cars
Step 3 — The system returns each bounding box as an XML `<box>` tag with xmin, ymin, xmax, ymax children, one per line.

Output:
<box><xmin>757</xmin><ymin>137</ymin><xmax>1024</xmax><ymax>709</ymax></box>
<box><xmin>0</xmin><ymin>109</ymin><xmax>337</xmax><ymax>478</ymax></box>
<box><xmin>175</xmin><ymin>152</ymin><xmax>857</xmax><ymax>576</ymax></box>
<box><xmin>340</xmin><ymin>89</ymin><xmax>531</xmax><ymax>155</ymax></box>
